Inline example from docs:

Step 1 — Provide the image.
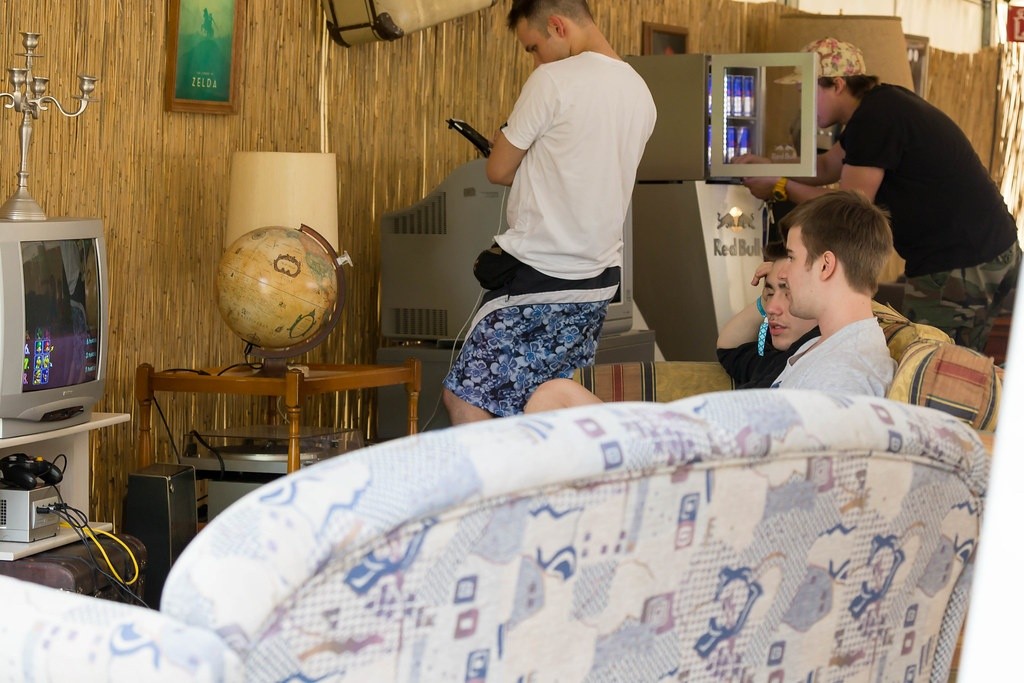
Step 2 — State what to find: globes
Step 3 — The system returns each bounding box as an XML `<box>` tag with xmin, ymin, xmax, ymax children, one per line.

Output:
<box><xmin>214</xmin><ymin>224</ymin><xmax>347</xmax><ymax>378</ymax></box>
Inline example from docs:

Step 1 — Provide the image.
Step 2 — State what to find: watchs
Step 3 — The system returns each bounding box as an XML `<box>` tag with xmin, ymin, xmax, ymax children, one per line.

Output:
<box><xmin>771</xmin><ymin>178</ymin><xmax>789</xmax><ymax>201</ymax></box>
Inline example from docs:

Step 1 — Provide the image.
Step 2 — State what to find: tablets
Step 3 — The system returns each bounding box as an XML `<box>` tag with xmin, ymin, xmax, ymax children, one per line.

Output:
<box><xmin>449</xmin><ymin>118</ymin><xmax>493</xmax><ymax>151</ymax></box>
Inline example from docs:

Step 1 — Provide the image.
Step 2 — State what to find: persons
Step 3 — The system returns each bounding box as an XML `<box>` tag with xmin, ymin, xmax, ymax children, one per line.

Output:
<box><xmin>717</xmin><ymin>240</ymin><xmax>821</xmax><ymax>390</ymax></box>
<box><xmin>730</xmin><ymin>35</ymin><xmax>1023</xmax><ymax>354</ymax></box>
<box><xmin>438</xmin><ymin>0</ymin><xmax>657</xmax><ymax>427</ymax></box>
<box><xmin>769</xmin><ymin>187</ymin><xmax>894</xmax><ymax>398</ymax></box>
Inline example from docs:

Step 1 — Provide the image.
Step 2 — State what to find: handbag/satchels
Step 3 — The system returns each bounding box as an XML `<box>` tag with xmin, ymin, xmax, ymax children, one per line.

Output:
<box><xmin>474</xmin><ymin>245</ymin><xmax>520</xmax><ymax>292</ymax></box>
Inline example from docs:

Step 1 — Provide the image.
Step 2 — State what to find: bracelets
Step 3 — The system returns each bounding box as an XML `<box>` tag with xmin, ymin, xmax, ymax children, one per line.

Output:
<box><xmin>756</xmin><ymin>295</ymin><xmax>768</xmax><ymax>357</ymax></box>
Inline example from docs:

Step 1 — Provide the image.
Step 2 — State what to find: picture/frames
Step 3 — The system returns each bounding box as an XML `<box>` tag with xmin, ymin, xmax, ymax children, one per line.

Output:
<box><xmin>165</xmin><ymin>0</ymin><xmax>247</xmax><ymax>115</ymax></box>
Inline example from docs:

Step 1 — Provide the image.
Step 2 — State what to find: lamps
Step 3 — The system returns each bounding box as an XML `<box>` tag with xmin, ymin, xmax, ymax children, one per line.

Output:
<box><xmin>777</xmin><ymin>13</ymin><xmax>914</xmax><ymax>93</ymax></box>
<box><xmin>226</xmin><ymin>151</ymin><xmax>339</xmax><ymax>377</ymax></box>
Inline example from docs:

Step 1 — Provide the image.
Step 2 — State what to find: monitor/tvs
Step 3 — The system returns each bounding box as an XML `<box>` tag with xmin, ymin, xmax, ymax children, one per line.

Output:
<box><xmin>1</xmin><ymin>217</ymin><xmax>111</xmax><ymax>422</ymax></box>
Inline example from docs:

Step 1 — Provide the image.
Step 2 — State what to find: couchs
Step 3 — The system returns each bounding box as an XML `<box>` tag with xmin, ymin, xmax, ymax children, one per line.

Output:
<box><xmin>0</xmin><ymin>301</ymin><xmax>1007</xmax><ymax>683</ymax></box>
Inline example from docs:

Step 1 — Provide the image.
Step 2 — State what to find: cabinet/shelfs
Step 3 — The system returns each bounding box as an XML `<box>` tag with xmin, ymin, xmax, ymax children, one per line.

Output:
<box><xmin>376</xmin><ymin>329</ymin><xmax>657</xmax><ymax>437</ymax></box>
<box><xmin>134</xmin><ymin>358</ymin><xmax>421</xmax><ymax>532</ymax></box>
<box><xmin>619</xmin><ymin>52</ymin><xmax>820</xmax><ymax>362</ymax></box>
<box><xmin>0</xmin><ymin>413</ymin><xmax>131</xmax><ymax>562</ymax></box>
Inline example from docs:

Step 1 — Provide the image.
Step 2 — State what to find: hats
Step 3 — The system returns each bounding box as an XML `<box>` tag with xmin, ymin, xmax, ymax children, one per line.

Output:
<box><xmin>772</xmin><ymin>37</ymin><xmax>867</xmax><ymax>86</ymax></box>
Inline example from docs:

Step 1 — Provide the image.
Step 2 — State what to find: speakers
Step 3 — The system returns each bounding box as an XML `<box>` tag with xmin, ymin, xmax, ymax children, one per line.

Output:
<box><xmin>121</xmin><ymin>463</ymin><xmax>197</xmax><ymax>610</ymax></box>
